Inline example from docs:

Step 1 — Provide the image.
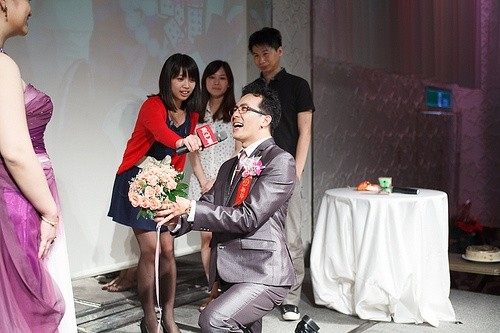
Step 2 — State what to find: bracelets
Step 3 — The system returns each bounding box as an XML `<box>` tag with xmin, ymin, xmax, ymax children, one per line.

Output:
<box><xmin>41</xmin><ymin>215</ymin><xmax>59</xmax><ymax>225</ymax></box>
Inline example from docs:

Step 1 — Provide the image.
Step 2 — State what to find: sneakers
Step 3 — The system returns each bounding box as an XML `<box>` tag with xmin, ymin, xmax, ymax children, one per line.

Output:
<box><xmin>283</xmin><ymin>306</ymin><xmax>300</xmax><ymax>320</ymax></box>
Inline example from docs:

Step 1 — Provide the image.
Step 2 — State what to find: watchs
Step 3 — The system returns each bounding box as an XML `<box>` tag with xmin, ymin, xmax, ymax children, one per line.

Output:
<box><xmin>185</xmin><ymin>200</ymin><xmax>192</xmax><ymax>216</ymax></box>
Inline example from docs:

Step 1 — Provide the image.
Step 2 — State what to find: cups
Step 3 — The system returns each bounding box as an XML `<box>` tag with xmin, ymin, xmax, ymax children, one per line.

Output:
<box><xmin>378</xmin><ymin>177</ymin><xmax>392</xmax><ymax>195</ymax></box>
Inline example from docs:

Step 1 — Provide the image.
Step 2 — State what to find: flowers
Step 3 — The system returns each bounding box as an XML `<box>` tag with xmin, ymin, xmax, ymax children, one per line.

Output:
<box><xmin>242</xmin><ymin>155</ymin><xmax>264</xmax><ymax>177</ymax></box>
<box><xmin>128</xmin><ymin>155</ymin><xmax>189</xmax><ymax>222</ymax></box>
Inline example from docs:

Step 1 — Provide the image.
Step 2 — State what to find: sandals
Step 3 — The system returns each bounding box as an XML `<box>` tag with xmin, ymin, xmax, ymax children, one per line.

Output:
<box><xmin>102</xmin><ymin>276</ymin><xmax>136</xmax><ymax>291</ymax></box>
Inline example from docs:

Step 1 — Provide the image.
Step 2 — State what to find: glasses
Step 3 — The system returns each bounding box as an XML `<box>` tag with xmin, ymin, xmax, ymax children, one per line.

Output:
<box><xmin>229</xmin><ymin>103</ymin><xmax>265</xmax><ymax>115</ymax></box>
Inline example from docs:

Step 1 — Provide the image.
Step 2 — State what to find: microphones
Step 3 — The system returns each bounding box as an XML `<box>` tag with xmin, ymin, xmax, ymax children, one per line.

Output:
<box><xmin>388</xmin><ymin>185</ymin><xmax>420</xmax><ymax>195</ymax></box>
<box><xmin>175</xmin><ymin>124</ymin><xmax>228</xmax><ymax>156</ymax></box>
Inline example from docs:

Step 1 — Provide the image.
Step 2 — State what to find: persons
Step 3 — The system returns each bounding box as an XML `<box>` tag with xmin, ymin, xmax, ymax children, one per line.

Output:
<box><xmin>101</xmin><ymin>267</ymin><xmax>136</xmax><ymax>292</ymax></box>
<box><xmin>154</xmin><ymin>87</ymin><xmax>295</xmax><ymax>333</ymax></box>
<box><xmin>0</xmin><ymin>0</ymin><xmax>77</xmax><ymax>333</ymax></box>
<box><xmin>188</xmin><ymin>60</ymin><xmax>242</xmax><ymax>312</ymax></box>
<box><xmin>107</xmin><ymin>53</ymin><xmax>202</xmax><ymax>333</ymax></box>
<box><xmin>241</xmin><ymin>27</ymin><xmax>315</xmax><ymax>320</ymax></box>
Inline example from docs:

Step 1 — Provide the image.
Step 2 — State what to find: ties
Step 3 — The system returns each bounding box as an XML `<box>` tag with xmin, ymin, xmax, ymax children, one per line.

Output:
<box><xmin>230</xmin><ymin>150</ymin><xmax>247</xmax><ymax>192</ymax></box>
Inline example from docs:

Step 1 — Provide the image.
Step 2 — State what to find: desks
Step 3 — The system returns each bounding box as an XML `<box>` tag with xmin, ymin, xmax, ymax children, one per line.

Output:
<box><xmin>310</xmin><ymin>187</ymin><xmax>457</xmax><ymax>328</ymax></box>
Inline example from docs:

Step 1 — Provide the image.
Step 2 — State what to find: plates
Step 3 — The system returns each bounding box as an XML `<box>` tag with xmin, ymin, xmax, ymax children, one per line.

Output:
<box><xmin>462</xmin><ymin>254</ymin><xmax>500</xmax><ymax>263</ymax></box>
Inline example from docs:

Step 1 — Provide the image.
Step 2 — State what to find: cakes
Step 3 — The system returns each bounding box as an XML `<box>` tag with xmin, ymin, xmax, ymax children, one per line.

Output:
<box><xmin>465</xmin><ymin>244</ymin><xmax>500</xmax><ymax>260</ymax></box>
